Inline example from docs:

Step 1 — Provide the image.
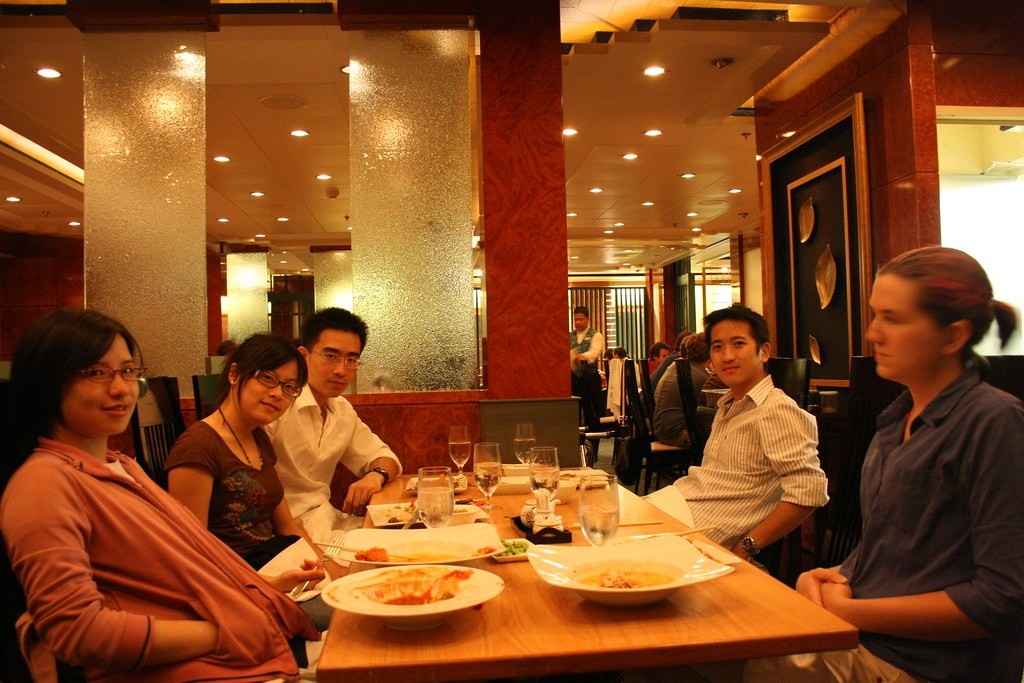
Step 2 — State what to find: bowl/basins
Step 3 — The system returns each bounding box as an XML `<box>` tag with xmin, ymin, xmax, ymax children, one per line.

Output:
<box><xmin>546</xmin><ymin>479</ymin><xmax>580</xmax><ymax>505</ymax></box>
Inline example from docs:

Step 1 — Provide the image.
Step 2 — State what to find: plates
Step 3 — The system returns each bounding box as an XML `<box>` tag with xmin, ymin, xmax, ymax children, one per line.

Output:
<box><xmin>527</xmin><ymin>534</ymin><xmax>735</xmax><ymax>609</ymax></box>
<box><xmin>321</xmin><ymin>564</ymin><xmax>505</xmax><ymax>632</ymax></box>
<box><xmin>400</xmin><ymin>472</ymin><xmax>467</xmax><ymax>495</ymax></box>
<box><xmin>494</xmin><ymin>475</ymin><xmax>533</xmax><ymax>495</ymax></box>
<box><xmin>629</xmin><ymin>535</ymin><xmax>741</xmax><ymax>565</ymax></box>
<box><xmin>340</xmin><ymin>522</ymin><xmax>507</xmax><ymax>566</ymax></box>
<box><xmin>365</xmin><ymin>500</ymin><xmax>486</xmax><ymax>526</ymax></box>
<box><xmin>492</xmin><ymin>537</ymin><xmax>532</xmax><ymax>562</ymax></box>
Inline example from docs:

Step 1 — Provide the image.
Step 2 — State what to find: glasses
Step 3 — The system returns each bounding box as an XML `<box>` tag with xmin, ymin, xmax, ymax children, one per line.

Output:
<box><xmin>79</xmin><ymin>366</ymin><xmax>148</xmax><ymax>382</ymax></box>
<box><xmin>250</xmin><ymin>369</ymin><xmax>302</xmax><ymax>398</ymax></box>
<box><xmin>308</xmin><ymin>350</ymin><xmax>362</xmax><ymax>369</ymax></box>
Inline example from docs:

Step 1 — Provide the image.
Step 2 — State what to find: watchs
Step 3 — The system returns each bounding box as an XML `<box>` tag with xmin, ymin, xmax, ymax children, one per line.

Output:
<box><xmin>741</xmin><ymin>534</ymin><xmax>760</xmax><ymax>555</ymax></box>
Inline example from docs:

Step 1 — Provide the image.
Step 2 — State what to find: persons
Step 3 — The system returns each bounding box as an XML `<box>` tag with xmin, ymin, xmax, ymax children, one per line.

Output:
<box><xmin>636</xmin><ymin>303</ymin><xmax>829</xmax><ymax>567</ymax></box>
<box><xmin>569</xmin><ymin>305</ymin><xmax>606</xmax><ymax>462</ymax></box>
<box><xmin>645</xmin><ymin>330</ymin><xmax>730</xmax><ymax>451</ymax></box>
<box><xmin>165</xmin><ymin>330</ymin><xmax>349</xmax><ymax>628</ymax></box>
<box><xmin>1</xmin><ymin>308</ymin><xmax>329</xmax><ymax>683</ymax></box>
<box><xmin>263</xmin><ymin>307</ymin><xmax>405</xmax><ymax>532</ymax></box>
<box><xmin>603</xmin><ymin>347</ymin><xmax>641</xmax><ymax>390</ymax></box>
<box><xmin>739</xmin><ymin>246</ymin><xmax>1024</xmax><ymax>683</ymax></box>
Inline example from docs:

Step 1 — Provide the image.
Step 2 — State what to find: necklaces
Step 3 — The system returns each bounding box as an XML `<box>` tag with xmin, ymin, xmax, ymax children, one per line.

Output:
<box><xmin>217</xmin><ymin>406</ymin><xmax>264</xmax><ymax>470</ymax></box>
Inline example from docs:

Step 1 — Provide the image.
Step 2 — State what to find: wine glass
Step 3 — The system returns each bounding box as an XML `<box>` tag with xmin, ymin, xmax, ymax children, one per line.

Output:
<box><xmin>526</xmin><ymin>444</ymin><xmax>560</xmax><ymax>505</ymax></box>
<box><xmin>513</xmin><ymin>423</ymin><xmax>538</xmax><ymax>467</ymax></box>
<box><xmin>473</xmin><ymin>441</ymin><xmax>503</xmax><ymax>510</ymax></box>
<box><xmin>417</xmin><ymin>466</ymin><xmax>454</xmax><ymax>527</ymax></box>
<box><xmin>580</xmin><ymin>473</ymin><xmax>620</xmax><ymax>549</ymax></box>
<box><xmin>447</xmin><ymin>425</ymin><xmax>472</xmax><ymax>478</ymax></box>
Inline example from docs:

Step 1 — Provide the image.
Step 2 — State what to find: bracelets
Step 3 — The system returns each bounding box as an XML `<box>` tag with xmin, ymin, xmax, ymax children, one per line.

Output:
<box><xmin>367</xmin><ymin>467</ymin><xmax>389</xmax><ymax>484</ymax></box>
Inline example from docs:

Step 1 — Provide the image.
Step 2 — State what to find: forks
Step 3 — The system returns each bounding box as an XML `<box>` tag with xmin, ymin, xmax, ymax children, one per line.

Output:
<box><xmin>290</xmin><ymin>535</ymin><xmax>344</xmax><ymax>597</ymax></box>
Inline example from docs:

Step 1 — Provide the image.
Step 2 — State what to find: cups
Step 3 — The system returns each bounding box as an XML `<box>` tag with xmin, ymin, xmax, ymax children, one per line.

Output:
<box><xmin>819</xmin><ymin>391</ymin><xmax>839</xmax><ymax>413</ymax></box>
